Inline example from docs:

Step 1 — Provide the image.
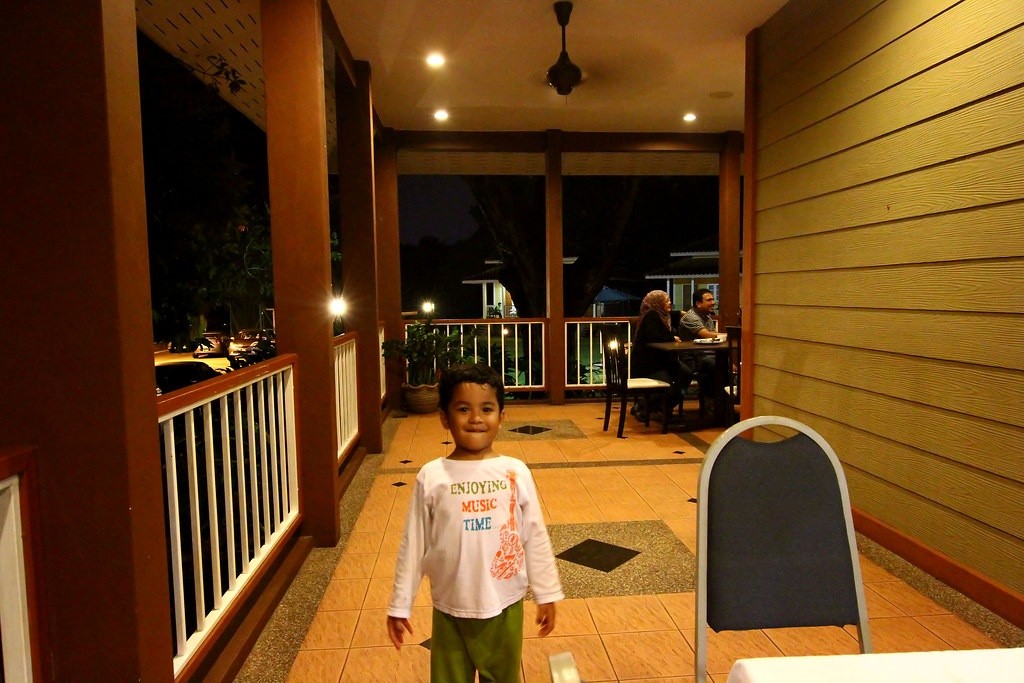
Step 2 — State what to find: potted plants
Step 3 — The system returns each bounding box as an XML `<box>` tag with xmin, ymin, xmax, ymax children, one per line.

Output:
<box><xmin>379</xmin><ymin>323</ymin><xmax>485</xmax><ymax>414</ymax></box>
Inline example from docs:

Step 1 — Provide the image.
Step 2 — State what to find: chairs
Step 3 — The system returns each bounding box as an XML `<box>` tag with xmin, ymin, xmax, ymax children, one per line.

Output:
<box><xmin>599</xmin><ymin>323</ymin><xmax>673</xmax><ymax>438</ymax></box>
<box><xmin>720</xmin><ymin>325</ymin><xmax>741</xmax><ymax>428</ymax></box>
<box><xmin>694</xmin><ymin>414</ymin><xmax>874</xmax><ymax>683</ymax></box>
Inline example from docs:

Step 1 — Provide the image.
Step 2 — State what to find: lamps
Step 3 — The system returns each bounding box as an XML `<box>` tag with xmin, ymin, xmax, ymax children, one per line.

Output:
<box><xmin>542</xmin><ymin>0</ymin><xmax>591</xmax><ymax>96</ymax></box>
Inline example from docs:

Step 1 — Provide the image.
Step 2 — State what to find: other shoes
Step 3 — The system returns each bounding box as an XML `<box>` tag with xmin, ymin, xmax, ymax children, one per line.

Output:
<box><xmin>657</xmin><ymin>408</ymin><xmax>676</xmax><ymax>418</ymax></box>
<box><xmin>630</xmin><ymin>407</ymin><xmax>646</xmax><ymax>423</ymax></box>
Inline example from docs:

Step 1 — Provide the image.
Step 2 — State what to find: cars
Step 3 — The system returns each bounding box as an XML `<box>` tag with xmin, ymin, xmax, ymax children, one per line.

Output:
<box><xmin>192</xmin><ymin>333</ymin><xmax>230</xmax><ymax>357</ymax></box>
<box><xmin>168</xmin><ymin>338</ymin><xmax>197</xmax><ymax>354</ymax></box>
<box><xmin>228</xmin><ymin>329</ymin><xmax>272</xmax><ymax>358</ymax></box>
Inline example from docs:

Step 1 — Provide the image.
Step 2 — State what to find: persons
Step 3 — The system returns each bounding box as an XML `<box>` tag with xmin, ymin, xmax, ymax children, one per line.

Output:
<box><xmin>630</xmin><ymin>288</ymin><xmax>733</xmax><ymax>422</ymax></box>
<box><xmin>387</xmin><ymin>363</ymin><xmax>565</xmax><ymax>683</ymax></box>
<box><xmin>509</xmin><ymin>304</ymin><xmax>517</xmax><ymax>317</ymax></box>
<box><xmin>494</xmin><ymin>302</ymin><xmax>502</xmax><ymax>318</ymax></box>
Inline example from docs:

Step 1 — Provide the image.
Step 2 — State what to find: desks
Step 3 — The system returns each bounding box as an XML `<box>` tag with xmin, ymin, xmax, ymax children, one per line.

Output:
<box><xmin>726</xmin><ymin>647</ymin><xmax>1024</xmax><ymax>683</ymax></box>
<box><xmin>649</xmin><ymin>341</ymin><xmax>738</xmax><ymax>425</ymax></box>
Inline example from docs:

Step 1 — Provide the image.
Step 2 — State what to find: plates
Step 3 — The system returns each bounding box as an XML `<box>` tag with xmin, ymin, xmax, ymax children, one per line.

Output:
<box><xmin>693</xmin><ymin>338</ymin><xmax>723</xmax><ymax>343</ymax></box>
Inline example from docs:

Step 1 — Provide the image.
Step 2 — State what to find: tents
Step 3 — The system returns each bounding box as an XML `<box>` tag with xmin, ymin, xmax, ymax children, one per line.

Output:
<box><xmin>591</xmin><ymin>285</ymin><xmax>642</xmax><ymax>318</ymax></box>
